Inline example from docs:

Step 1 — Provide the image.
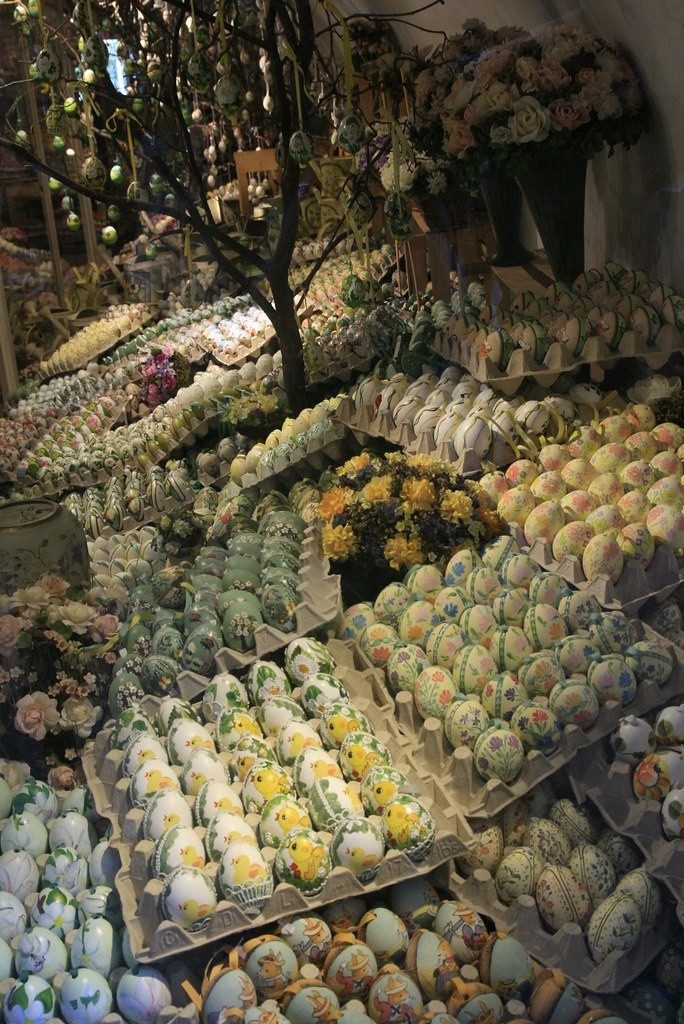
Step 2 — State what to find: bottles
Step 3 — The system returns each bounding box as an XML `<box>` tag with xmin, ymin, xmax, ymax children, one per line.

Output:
<box><xmin>0</xmin><ymin>499</ymin><xmax>92</xmax><ymax>601</ymax></box>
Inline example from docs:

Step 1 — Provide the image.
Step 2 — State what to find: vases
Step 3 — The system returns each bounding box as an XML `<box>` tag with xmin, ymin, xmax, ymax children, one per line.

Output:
<box><xmin>237</xmin><ymin>424</ymin><xmax>272</xmax><ymax>442</ymax></box>
<box><xmin>482</xmin><ymin>168</ymin><xmax>538</xmax><ymax>267</ymax></box>
<box><xmin>513</xmin><ymin>141</ymin><xmax>587</xmax><ymax>286</ymax></box>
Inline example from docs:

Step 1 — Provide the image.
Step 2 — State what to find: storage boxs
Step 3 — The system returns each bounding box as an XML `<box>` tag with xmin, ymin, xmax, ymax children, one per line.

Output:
<box><xmin>234</xmin><ymin>149</ymin><xmax>284</xmax><ymax>216</ymax></box>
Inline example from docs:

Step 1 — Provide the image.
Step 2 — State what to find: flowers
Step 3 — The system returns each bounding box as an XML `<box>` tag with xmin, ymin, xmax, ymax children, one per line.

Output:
<box><xmin>359</xmin><ymin>17</ymin><xmax>647</xmax><ymax>194</ymax></box>
<box><xmin>137</xmin><ymin>346</ymin><xmax>192</xmax><ymax>407</ymax></box>
<box><xmin>224</xmin><ymin>391</ymin><xmax>285</xmax><ymax>428</ymax></box>
<box><xmin>0</xmin><ymin>563</ymin><xmax>122</xmax><ymax>829</ymax></box>
<box><xmin>155</xmin><ymin>505</ymin><xmax>210</xmax><ymax>555</ymax></box>
<box><xmin>313</xmin><ymin>446</ymin><xmax>499</xmax><ymax>575</ymax></box>
